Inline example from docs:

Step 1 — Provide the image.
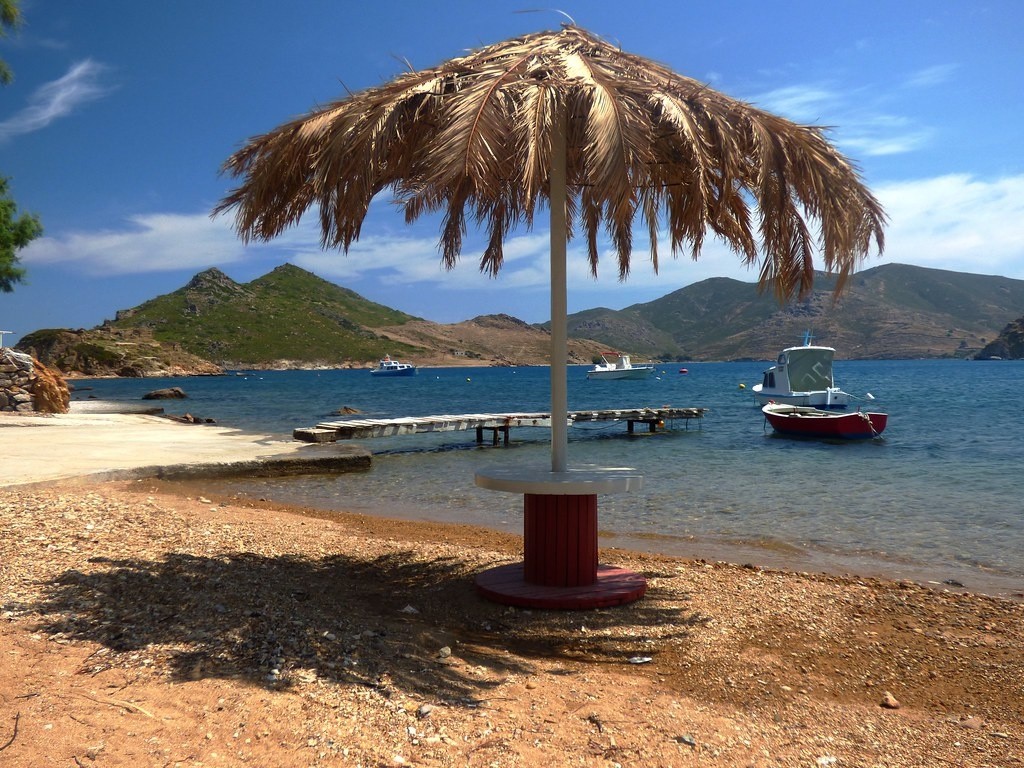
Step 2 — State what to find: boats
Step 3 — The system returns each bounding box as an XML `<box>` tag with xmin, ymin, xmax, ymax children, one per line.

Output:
<box><xmin>751</xmin><ymin>330</ymin><xmax>849</xmax><ymax>410</ymax></box>
<box><xmin>370</xmin><ymin>353</ymin><xmax>417</xmax><ymax>377</ymax></box>
<box><xmin>762</xmin><ymin>400</ymin><xmax>888</xmax><ymax>441</ymax></box>
<box><xmin>586</xmin><ymin>349</ymin><xmax>655</xmax><ymax>380</ymax></box>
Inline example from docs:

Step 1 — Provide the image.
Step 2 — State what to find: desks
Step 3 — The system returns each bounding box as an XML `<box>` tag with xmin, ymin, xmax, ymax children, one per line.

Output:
<box><xmin>472</xmin><ymin>464</ymin><xmax>647</xmax><ymax>611</ymax></box>
